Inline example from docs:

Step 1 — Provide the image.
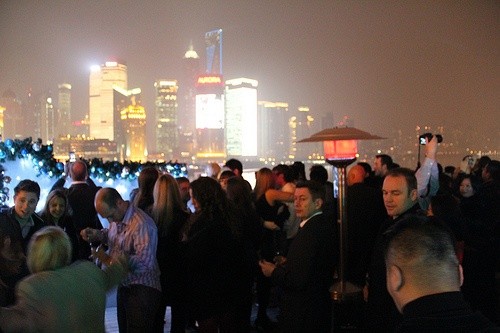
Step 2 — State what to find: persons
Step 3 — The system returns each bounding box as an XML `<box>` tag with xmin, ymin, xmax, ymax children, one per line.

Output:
<box><xmin>50</xmin><ymin>160</ymin><xmax>109</xmax><ymax>264</ymax></box>
<box><xmin>79</xmin><ymin>187</ymin><xmax>164</xmax><ymax>333</ymax></box>
<box><xmin>131</xmin><ymin>137</ymin><xmax>500</xmax><ymax>333</ymax></box>
<box><xmin>0</xmin><ymin>179</ymin><xmax>49</xmax><ymax>308</ymax></box>
<box><xmin>0</xmin><ymin>134</ymin><xmax>134</xmax><ymax>207</ymax></box>
<box><xmin>0</xmin><ymin>225</ymin><xmax>127</xmax><ymax>333</ymax></box>
<box><xmin>39</xmin><ymin>188</ymin><xmax>80</xmax><ymax>265</ymax></box>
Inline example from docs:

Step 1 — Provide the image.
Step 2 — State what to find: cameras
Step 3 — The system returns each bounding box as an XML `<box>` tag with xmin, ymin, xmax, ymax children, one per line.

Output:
<box><xmin>419</xmin><ymin>133</ymin><xmax>442</xmax><ymax>145</ymax></box>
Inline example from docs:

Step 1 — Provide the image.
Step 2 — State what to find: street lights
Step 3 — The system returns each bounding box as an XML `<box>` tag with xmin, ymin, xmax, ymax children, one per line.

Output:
<box><xmin>296</xmin><ymin>126</ymin><xmax>389</xmax><ymax>305</ymax></box>
